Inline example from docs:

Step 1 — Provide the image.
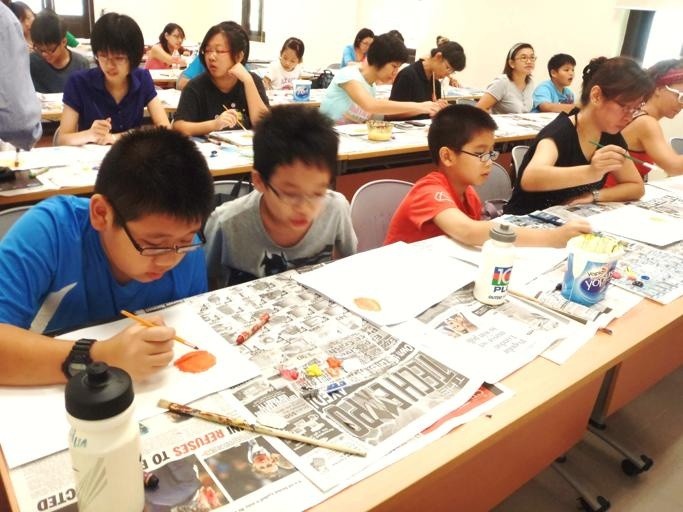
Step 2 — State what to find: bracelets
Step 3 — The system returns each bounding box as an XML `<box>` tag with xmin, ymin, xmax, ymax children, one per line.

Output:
<box><xmin>589</xmin><ymin>188</ymin><xmax>601</xmax><ymax>202</ymax></box>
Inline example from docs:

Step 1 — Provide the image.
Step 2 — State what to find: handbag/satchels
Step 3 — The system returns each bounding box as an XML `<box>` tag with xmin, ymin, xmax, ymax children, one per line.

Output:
<box><xmin>481</xmin><ymin>199</ymin><xmax>508</xmax><ymax>220</ymax></box>
<box><xmin>317</xmin><ymin>70</ymin><xmax>334</xmax><ymax>88</ymax></box>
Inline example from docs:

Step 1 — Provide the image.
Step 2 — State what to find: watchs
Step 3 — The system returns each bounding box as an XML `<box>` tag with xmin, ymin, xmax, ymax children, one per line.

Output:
<box><xmin>62</xmin><ymin>338</ymin><xmax>95</xmax><ymax>380</ymax></box>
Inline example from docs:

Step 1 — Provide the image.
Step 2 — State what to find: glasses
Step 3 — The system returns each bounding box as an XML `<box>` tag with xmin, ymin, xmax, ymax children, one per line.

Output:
<box><xmin>94</xmin><ymin>53</ymin><xmax>127</xmax><ymax>64</ymax></box>
<box><xmin>613</xmin><ymin>84</ymin><xmax>683</xmax><ymax>114</ymax></box>
<box><xmin>445</xmin><ymin>62</ymin><xmax>455</xmax><ymax>76</ymax></box>
<box><xmin>517</xmin><ymin>54</ymin><xmax>536</xmax><ymax>62</ymax></box>
<box><xmin>33</xmin><ymin>42</ymin><xmax>60</xmax><ymax>54</ymax></box>
<box><xmin>109</xmin><ymin>182</ymin><xmax>326</xmax><ymax>256</ymax></box>
<box><xmin>452</xmin><ymin>147</ymin><xmax>499</xmax><ymax>162</ymax></box>
<box><xmin>201</xmin><ymin>46</ymin><xmax>232</xmax><ymax>54</ymax></box>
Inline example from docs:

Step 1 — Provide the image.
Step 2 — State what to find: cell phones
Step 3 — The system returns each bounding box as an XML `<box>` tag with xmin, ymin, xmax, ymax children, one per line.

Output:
<box><xmin>529</xmin><ymin>210</ymin><xmax>567</xmax><ymax>226</ymax></box>
<box><xmin>406</xmin><ymin>120</ymin><xmax>425</xmax><ymax>127</ymax></box>
<box><xmin>393</xmin><ymin>121</ymin><xmax>414</xmax><ymax>130</ymax></box>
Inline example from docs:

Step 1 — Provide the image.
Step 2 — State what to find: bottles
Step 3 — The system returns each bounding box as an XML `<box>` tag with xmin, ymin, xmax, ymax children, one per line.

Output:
<box><xmin>474</xmin><ymin>222</ymin><xmax>516</xmax><ymax>304</ymax></box>
<box><xmin>65</xmin><ymin>361</ymin><xmax>144</xmax><ymax>511</ymax></box>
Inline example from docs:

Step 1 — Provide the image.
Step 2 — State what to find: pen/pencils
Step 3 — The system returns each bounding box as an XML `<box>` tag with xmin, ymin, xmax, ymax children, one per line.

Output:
<box><xmin>29</xmin><ymin>167</ymin><xmax>49</xmax><ymax>178</ymax></box>
<box><xmin>235</xmin><ymin>313</ymin><xmax>268</xmax><ymax>344</ymax></box>
<box><xmin>144</xmin><ymin>472</ymin><xmax>159</xmax><ymax>488</ymax></box>
<box><xmin>207</xmin><ymin>138</ymin><xmax>221</xmax><ymax>145</ymax></box>
<box><xmin>120</xmin><ymin>310</ymin><xmax>200</xmax><ymax>352</ymax></box>
<box><xmin>15</xmin><ymin>148</ymin><xmax>19</xmax><ymax>167</ymax></box>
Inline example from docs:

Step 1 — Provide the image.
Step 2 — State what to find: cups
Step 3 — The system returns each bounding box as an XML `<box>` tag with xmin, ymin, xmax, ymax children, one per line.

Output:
<box><xmin>560</xmin><ymin>235</ymin><xmax>623</xmax><ymax>306</ymax></box>
<box><xmin>291</xmin><ymin>79</ymin><xmax>313</xmax><ymax>102</ymax></box>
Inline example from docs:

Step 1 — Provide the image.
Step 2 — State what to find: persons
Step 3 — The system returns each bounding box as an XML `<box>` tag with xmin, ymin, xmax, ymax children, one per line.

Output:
<box><xmin>601</xmin><ymin>60</ymin><xmax>683</xmax><ymax>189</ymax></box>
<box><xmin>319</xmin><ymin>27</ymin><xmax>465</xmax><ymax>124</ymax></box>
<box><xmin>384</xmin><ymin>104</ymin><xmax>592</xmax><ymax>249</ymax></box>
<box><xmin>0</xmin><ymin>125</ymin><xmax>216</xmax><ymax>385</ymax></box>
<box><xmin>475</xmin><ymin>42</ymin><xmax>537</xmax><ymax>114</ymax></box>
<box><xmin>531</xmin><ymin>53</ymin><xmax>582</xmax><ymax>113</ymax></box>
<box><xmin>173</xmin><ymin>20</ymin><xmax>270</xmax><ymax>135</ymax></box>
<box><xmin>263</xmin><ymin>37</ymin><xmax>304</xmax><ymax>89</ymax></box>
<box><xmin>55</xmin><ymin>12</ymin><xmax>172</xmax><ymax>144</ymax></box>
<box><xmin>1</xmin><ymin>0</ymin><xmax>90</xmax><ymax>150</ymax></box>
<box><xmin>502</xmin><ymin>56</ymin><xmax>656</xmax><ymax>215</ymax></box>
<box><xmin>144</xmin><ymin>23</ymin><xmax>186</xmax><ymax>70</ymax></box>
<box><xmin>203</xmin><ymin>103</ymin><xmax>358</xmax><ymax>290</ymax></box>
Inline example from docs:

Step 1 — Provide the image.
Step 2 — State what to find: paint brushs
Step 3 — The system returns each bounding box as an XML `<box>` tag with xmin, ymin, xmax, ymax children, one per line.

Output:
<box><xmin>588</xmin><ymin>138</ymin><xmax>665</xmax><ymax>175</ymax></box>
<box><xmin>432</xmin><ymin>72</ymin><xmax>436</xmax><ymax>102</ymax></box>
<box><xmin>222</xmin><ymin>103</ymin><xmax>248</xmax><ymax>134</ymax></box>
<box><xmin>156</xmin><ymin>398</ymin><xmax>367</xmax><ymax>458</ymax></box>
<box><xmin>527</xmin><ymin>212</ymin><xmax>562</xmax><ymax>227</ymax></box>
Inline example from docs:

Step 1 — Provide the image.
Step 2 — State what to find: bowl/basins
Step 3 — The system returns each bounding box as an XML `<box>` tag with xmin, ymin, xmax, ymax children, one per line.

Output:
<box><xmin>366</xmin><ymin>119</ymin><xmax>394</xmax><ymax>141</ymax></box>
